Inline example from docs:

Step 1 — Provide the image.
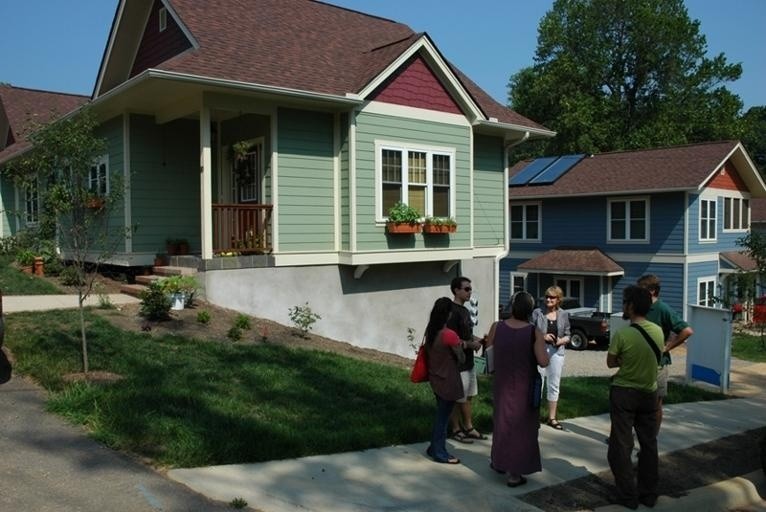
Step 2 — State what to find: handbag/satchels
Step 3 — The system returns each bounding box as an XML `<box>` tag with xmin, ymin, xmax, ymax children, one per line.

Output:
<box><xmin>410</xmin><ymin>342</ymin><xmax>428</xmax><ymax>384</ymax></box>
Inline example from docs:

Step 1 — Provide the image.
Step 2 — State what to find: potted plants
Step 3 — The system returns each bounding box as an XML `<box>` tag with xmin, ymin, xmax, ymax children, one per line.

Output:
<box><xmin>16</xmin><ymin>240</ymin><xmax>54</xmax><ymax>277</ymax></box>
<box><xmin>176</xmin><ymin>239</ymin><xmax>189</xmax><ymax>255</ymax></box>
<box><xmin>423</xmin><ymin>216</ymin><xmax>457</xmax><ymax>233</ymax></box>
<box><xmin>154</xmin><ymin>273</ymin><xmax>201</xmax><ymax>312</ymax></box>
<box><xmin>154</xmin><ymin>254</ymin><xmax>168</xmax><ymax>266</ymax></box>
<box><xmin>385</xmin><ymin>201</ymin><xmax>422</xmax><ymax>233</ymax></box>
<box><xmin>165</xmin><ymin>235</ymin><xmax>178</xmax><ymax>254</ymax></box>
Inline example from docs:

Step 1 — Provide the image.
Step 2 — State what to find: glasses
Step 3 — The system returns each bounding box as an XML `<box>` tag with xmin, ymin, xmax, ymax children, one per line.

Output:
<box><xmin>460</xmin><ymin>286</ymin><xmax>471</xmax><ymax>292</ymax></box>
<box><xmin>545</xmin><ymin>294</ymin><xmax>557</xmax><ymax>300</ymax></box>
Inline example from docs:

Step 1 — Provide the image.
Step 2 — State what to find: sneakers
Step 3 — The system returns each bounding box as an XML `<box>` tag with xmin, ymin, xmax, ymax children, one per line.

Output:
<box><xmin>434</xmin><ymin>455</ymin><xmax>460</xmax><ymax>464</ymax></box>
<box><xmin>636</xmin><ymin>495</ymin><xmax>657</xmax><ymax>509</ymax></box>
<box><xmin>608</xmin><ymin>491</ymin><xmax>638</xmax><ymax>510</ymax></box>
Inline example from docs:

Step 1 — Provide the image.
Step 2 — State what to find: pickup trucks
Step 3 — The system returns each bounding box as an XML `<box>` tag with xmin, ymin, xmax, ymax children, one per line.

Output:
<box><xmin>729</xmin><ymin>294</ymin><xmax>765</xmax><ymax>326</ymax></box>
<box><xmin>531</xmin><ymin>295</ymin><xmax>614</xmax><ymax>350</ymax></box>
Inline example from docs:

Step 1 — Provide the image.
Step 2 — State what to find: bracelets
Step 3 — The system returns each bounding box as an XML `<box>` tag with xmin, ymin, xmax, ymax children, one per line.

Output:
<box><xmin>463</xmin><ymin>340</ymin><xmax>468</xmax><ymax>349</ymax></box>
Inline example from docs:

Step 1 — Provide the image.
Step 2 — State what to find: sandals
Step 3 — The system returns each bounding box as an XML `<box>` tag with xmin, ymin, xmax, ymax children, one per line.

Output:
<box><xmin>546</xmin><ymin>416</ymin><xmax>563</xmax><ymax>430</ymax></box>
<box><xmin>451</xmin><ymin>430</ymin><xmax>474</xmax><ymax>444</ymax></box>
<box><xmin>463</xmin><ymin>427</ymin><xmax>488</xmax><ymax>440</ymax></box>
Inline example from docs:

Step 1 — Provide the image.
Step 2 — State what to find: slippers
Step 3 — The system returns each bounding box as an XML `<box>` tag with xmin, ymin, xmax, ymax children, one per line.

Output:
<box><xmin>489</xmin><ymin>462</ymin><xmax>506</xmax><ymax>475</ymax></box>
<box><xmin>506</xmin><ymin>475</ymin><xmax>527</xmax><ymax>488</ymax></box>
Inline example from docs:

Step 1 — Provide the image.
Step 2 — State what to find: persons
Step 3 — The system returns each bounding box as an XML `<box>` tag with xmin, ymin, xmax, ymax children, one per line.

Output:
<box><xmin>446</xmin><ymin>277</ymin><xmax>489</xmax><ymax>444</ymax></box>
<box><xmin>488</xmin><ymin>290</ymin><xmax>550</xmax><ymax>488</ymax></box>
<box><xmin>423</xmin><ymin>298</ymin><xmax>466</xmax><ymax>464</ymax></box>
<box><xmin>529</xmin><ymin>284</ymin><xmax>570</xmax><ymax>429</ymax></box>
<box><xmin>635</xmin><ymin>275</ymin><xmax>693</xmax><ymax>460</ymax></box>
<box><xmin>607</xmin><ymin>285</ymin><xmax>663</xmax><ymax>509</ymax></box>
